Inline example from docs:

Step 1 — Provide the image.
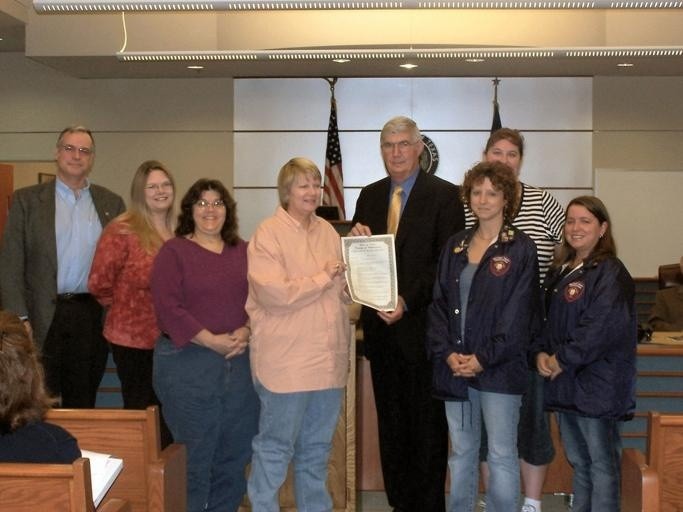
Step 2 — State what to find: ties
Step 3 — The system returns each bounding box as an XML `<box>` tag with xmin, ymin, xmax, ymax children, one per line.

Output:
<box><xmin>387</xmin><ymin>186</ymin><xmax>404</xmax><ymax>239</ymax></box>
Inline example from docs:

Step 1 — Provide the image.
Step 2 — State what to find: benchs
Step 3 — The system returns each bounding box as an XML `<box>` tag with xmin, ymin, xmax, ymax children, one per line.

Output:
<box><xmin>0</xmin><ymin>450</ymin><xmax>126</xmax><ymax>512</ymax></box>
<box><xmin>617</xmin><ymin>412</ymin><xmax>682</xmax><ymax>510</ymax></box>
<box><xmin>0</xmin><ymin>395</ymin><xmax>189</xmax><ymax>512</ymax></box>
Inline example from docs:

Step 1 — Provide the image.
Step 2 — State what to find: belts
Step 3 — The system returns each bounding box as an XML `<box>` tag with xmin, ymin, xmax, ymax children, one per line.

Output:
<box><xmin>59</xmin><ymin>293</ymin><xmax>92</xmax><ymax>300</ymax></box>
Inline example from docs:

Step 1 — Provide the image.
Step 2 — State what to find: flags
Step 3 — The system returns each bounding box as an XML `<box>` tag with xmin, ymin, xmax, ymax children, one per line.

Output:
<box><xmin>490</xmin><ymin>102</ymin><xmax>501</xmax><ymax>134</ymax></box>
<box><xmin>321</xmin><ymin>97</ymin><xmax>346</xmax><ymax>221</ymax></box>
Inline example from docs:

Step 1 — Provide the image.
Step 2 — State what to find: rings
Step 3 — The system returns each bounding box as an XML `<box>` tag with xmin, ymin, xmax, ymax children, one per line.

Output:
<box><xmin>335</xmin><ymin>264</ymin><xmax>340</xmax><ymax>268</ymax></box>
<box><xmin>334</xmin><ymin>269</ymin><xmax>339</xmax><ymax>273</ymax></box>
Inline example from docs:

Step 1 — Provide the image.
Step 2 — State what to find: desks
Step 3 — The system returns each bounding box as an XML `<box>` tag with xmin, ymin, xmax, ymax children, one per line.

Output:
<box><xmin>627</xmin><ymin>328</ymin><xmax>682</xmax><ymax>454</ymax></box>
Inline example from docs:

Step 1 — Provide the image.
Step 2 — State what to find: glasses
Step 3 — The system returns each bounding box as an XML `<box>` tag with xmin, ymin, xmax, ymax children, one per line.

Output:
<box><xmin>58</xmin><ymin>142</ymin><xmax>91</xmax><ymax>156</ymax></box>
<box><xmin>196</xmin><ymin>199</ymin><xmax>226</xmax><ymax>210</ymax></box>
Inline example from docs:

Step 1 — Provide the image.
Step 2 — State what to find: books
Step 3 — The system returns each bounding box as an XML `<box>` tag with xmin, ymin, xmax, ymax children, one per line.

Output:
<box><xmin>667</xmin><ymin>333</ymin><xmax>682</xmax><ymax>343</ymax></box>
<box><xmin>79</xmin><ymin>449</ymin><xmax>125</xmax><ymax>511</ymax></box>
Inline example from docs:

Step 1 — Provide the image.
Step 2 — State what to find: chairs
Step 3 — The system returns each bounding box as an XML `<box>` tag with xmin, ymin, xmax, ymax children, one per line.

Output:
<box><xmin>657</xmin><ymin>263</ymin><xmax>682</xmax><ymax>294</ymax></box>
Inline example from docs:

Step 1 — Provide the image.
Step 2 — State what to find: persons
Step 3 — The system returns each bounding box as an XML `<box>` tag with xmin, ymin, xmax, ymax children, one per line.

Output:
<box><xmin>423</xmin><ymin>160</ymin><xmax>541</xmax><ymax>512</ymax></box>
<box><xmin>87</xmin><ymin>159</ymin><xmax>178</xmax><ymax>452</ymax></box>
<box><xmin>0</xmin><ymin>310</ymin><xmax>97</xmax><ymax>512</ymax></box>
<box><xmin>525</xmin><ymin>194</ymin><xmax>636</xmax><ymax>510</ymax></box>
<box><xmin>346</xmin><ymin>115</ymin><xmax>467</xmax><ymax>512</ymax></box>
<box><xmin>646</xmin><ymin>254</ymin><xmax>682</xmax><ymax>332</ymax></box>
<box><xmin>1</xmin><ymin>124</ymin><xmax>128</xmax><ymax>409</ymax></box>
<box><xmin>244</xmin><ymin>156</ymin><xmax>355</xmax><ymax>512</ymax></box>
<box><xmin>149</xmin><ymin>177</ymin><xmax>262</xmax><ymax>512</ymax></box>
<box><xmin>460</xmin><ymin>126</ymin><xmax>565</xmax><ymax>512</ymax></box>
